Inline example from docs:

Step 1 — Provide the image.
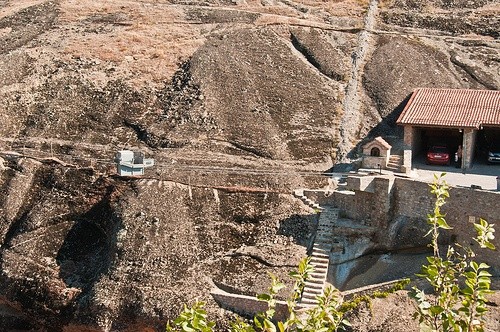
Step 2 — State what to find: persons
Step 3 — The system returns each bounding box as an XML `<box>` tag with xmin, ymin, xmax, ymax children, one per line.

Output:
<box><xmin>455</xmin><ymin>143</ymin><xmax>463</xmax><ymax>168</ymax></box>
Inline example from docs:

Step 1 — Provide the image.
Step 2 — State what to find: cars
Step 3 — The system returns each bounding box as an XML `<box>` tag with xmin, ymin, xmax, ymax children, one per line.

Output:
<box><xmin>486</xmin><ymin>144</ymin><xmax>500</xmax><ymax>164</ymax></box>
<box><xmin>427</xmin><ymin>145</ymin><xmax>450</xmax><ymax>166</ymax></box>
